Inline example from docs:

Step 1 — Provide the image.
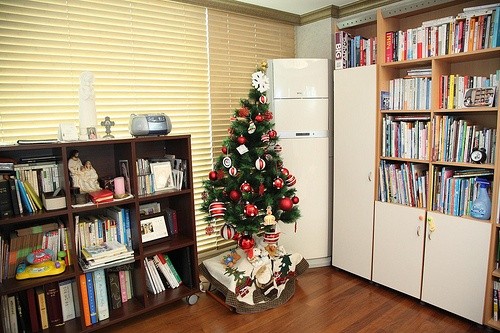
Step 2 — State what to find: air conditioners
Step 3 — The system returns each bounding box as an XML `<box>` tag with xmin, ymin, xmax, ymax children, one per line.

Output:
<box><xmin>262</xmin><ymin>57</ymin><xmax>333</xmax><ymax>270</ymax></box>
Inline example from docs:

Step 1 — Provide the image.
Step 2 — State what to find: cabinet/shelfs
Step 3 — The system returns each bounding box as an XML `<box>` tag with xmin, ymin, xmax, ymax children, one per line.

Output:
<box><xmin>0</xmin><ymin>134</ymin><xmax>199</xmax><ymax>333</ymax></box>
<box><xmin>331</xmin><ymin>0</ymin><xmax>500</xmax><ymax>330</ymax></box>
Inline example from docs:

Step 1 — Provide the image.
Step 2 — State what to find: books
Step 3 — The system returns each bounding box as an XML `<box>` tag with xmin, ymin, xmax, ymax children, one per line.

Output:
<box><xmin>17</xmin><ymin>139</ymin><xmax>61</xmax><ymax>144</ymax></box>
<box><xmin>431</xmin><ymin>116</ymin><xmax>496</xmax><ymax>165</ymax></box>
<box><xmin>385</xmin><ymin>3</ymin><xmax>500</xmax><ymax>63</ymax></box>
<box><xmin>388</xmin><ymin>67</ymin><xmax>500</xmax><ymax>110</ymax></box>
<box><xmin>430</xmin><ymin>166</ymin><xmax>494</xmax><ymax>216</ymax></box>
<box><xmin>494</xmin><ymin>196</ymin><xmax>500</xmax><ymax>323</ymax></box>
<box><xmin>135</xmin><ymin>155</ymin><xmax>188</xmax><ymax>198</ymax></box>
<box><xmin>0</xmin><ymin>153</ymin><xmax>67</xmax><ymax>216</ymax></box>
<box><xmin>382</xmin><ymin>115</ymin><xmax>430</xmax><ymax>164</ymax></box>
<box><xmin>335</xmin><ymin>31</ymin><xmax>376</xmax><ymax>70</ymax></box>
<box><xmin>378</xmin><ymin>160</ymin><xmax>429</xmax><ymax>208</ymax></box>
<box><xmin>0</xmin><ymin>202</ymin><xmax>183</xmax><ymax>333</ymax></box>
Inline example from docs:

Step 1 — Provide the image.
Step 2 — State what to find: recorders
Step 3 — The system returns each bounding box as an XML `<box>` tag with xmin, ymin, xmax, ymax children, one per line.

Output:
<box><xmin>130</xmin><ymin>113</ymin><xmax>171</xmax><ymax>136</ymax></box>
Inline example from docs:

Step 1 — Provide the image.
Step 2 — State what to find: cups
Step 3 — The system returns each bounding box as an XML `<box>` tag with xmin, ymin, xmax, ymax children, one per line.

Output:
<box><xmin>114</xmin><ymin>177</ymin><xmax>125</xmax><ymax>195</ymax></box>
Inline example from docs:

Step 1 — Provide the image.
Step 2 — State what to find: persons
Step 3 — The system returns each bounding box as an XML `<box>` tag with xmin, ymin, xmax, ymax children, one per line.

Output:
<box><xmin>383</xmin><ymin>98</ymin><xmax>389</xmax><ymax>106</ymax></box>
<box><xmin>69</xmin><ymin>150</ymin><xmax>101</xmax><ymax>193</ymax></box>
<box><xmin>487</xmin><ymin>93</ymin><xmax>494</xmax><ymax>106</ymax></box>
<box><xmin>479</xmin><ymin>98</ymin><xmax>486</xmax><ymax>105</ymax></box>
<box><xmin>474</xmin><ymin>98</ymin><xmax>479</xmax><ymax>105</ymax></box>
<box><xmin>464</xmin><ymin>96</ymin><xmax>472</xmax><ymax>106</ymax></box>
<box><xmin>89</xmin><ymin>130</ymin><xmax>95</xmax><ymax>138</ymax></box>
